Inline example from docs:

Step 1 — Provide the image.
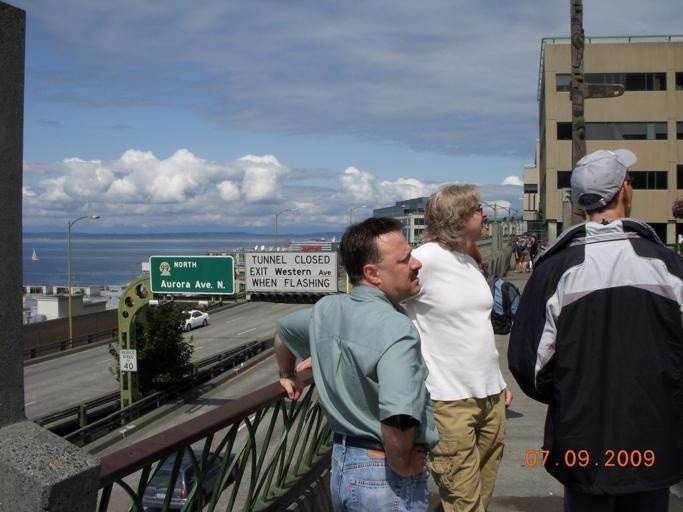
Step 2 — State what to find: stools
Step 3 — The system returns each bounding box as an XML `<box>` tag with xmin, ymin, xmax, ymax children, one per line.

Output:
<box><xmin>333</xmin><ymin>432</ymin><xmax>385</xmax><ymax>452</ymax></box>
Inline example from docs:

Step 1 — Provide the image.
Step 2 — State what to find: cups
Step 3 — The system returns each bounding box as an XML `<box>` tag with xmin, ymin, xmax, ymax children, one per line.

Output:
<box><xmin>471</xmin><ymin>204</ymin><xmax>482</xmax><ymax>216</ymax></box>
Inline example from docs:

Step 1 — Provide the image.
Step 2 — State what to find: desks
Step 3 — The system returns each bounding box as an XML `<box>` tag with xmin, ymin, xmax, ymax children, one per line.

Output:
<box><xmin>67</xmin><ymin>214</ymin><xmax>100</xmax><ymax>349</ymax></box>
<box><xmin>274</xmin><ymin>207</ymin><xmax>298</xmax><ymax>253</ymax></box>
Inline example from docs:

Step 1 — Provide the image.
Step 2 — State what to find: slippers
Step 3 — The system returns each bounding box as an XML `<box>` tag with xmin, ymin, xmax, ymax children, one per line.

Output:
<box><xmin>517</xmin><ymin>239</ymin><xmax>527</xmax><ymax>251</ymax></box>
<box><xmin>483</xmin><ymin>268</ymin><xmax>521</xmax><ymax>335</ymax></box>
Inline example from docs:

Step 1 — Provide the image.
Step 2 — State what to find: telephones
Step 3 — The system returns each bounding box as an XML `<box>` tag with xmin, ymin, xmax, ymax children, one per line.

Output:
<box><xmin>149</xmin><ymin>255</ymin><xmax>236</xmax><ymax>296</ymax></box>
<box><xmin>243</xmin><ymin>251</ymin><xmax>337</xmax><ymax>293</ymax></box>
<box><xmin>119</xmin><ymin>349</ymin><xmax>137</xmax><ymax>372</ymax></box>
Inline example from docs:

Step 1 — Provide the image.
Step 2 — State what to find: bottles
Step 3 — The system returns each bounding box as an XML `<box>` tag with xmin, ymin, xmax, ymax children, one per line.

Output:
<box><xmin>570</xmin><ymin>148</ymin><xmax>637</xmax><ymax>210</ymax></box>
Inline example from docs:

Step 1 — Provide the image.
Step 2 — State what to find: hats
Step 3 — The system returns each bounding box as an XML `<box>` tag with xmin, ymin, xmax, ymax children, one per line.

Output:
<box><xmin>178</xmin><ymin>310</ymin><xmax>208</xmax><ymax>332</ymax></box>
<box><xmin>141</xmin><ymin>449</ymin><xmax>240</xmax><ymax>512</ymax></box>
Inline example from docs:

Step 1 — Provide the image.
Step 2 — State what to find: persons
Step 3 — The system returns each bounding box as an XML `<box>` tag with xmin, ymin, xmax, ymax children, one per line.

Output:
<box><xmin>296</xmin><ymin>185</ymin><xmax>513</xmax><ymax>512</ymax></box>
<box><xmin>513</xmin><ymin>232</ymin><xmax>539</xmax><ymax>273</ymax></box>
<box><xmin>506</xmin><ymin>149</ymin><xmax>683</xmax><ymax>512</ymax></box>
<box><xmin>273</xmin><ymin>218</ymin><xmax>440</xmax><ymax>512</ymax></box>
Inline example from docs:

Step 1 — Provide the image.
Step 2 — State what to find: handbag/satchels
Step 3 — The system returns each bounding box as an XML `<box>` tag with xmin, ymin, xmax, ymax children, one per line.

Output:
<box><xmin>279</xmin><ymin>370</ymin><xmax>297</xmax><ymax>378</ymax></box>
